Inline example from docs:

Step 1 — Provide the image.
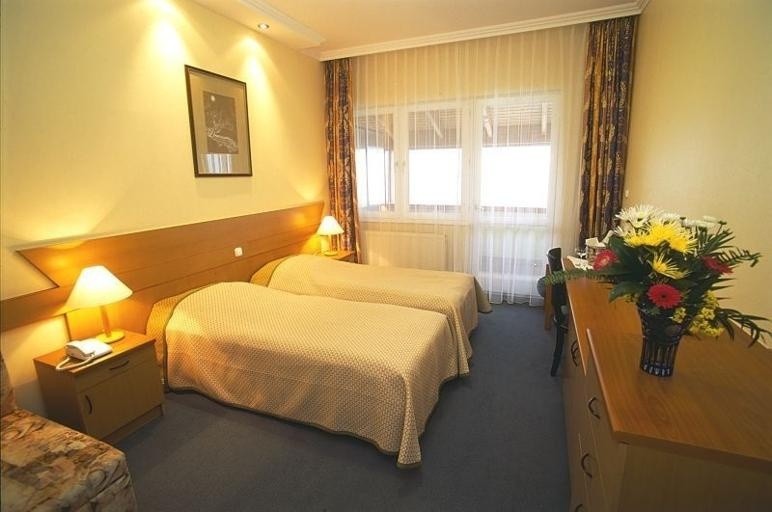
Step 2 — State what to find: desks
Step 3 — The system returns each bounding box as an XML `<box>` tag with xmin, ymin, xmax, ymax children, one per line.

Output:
<box><xmin>563</xmin><ymin>258</ymin><xmax>594</xmax><ymax>271</ymax></box>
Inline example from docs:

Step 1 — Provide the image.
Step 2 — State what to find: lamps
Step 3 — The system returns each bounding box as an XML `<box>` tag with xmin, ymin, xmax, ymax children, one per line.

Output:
<box><xmin>66</xmin><ymin>265</ymin><xmax>133</xmax><ymax>343</ymax></box>
<box><xmin>316</xmin><ymin>215</ymin><xmax>345</xmax><ymax>256</ymax></box>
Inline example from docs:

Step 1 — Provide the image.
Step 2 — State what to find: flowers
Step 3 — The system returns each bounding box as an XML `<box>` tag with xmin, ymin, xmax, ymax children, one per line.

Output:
<box><xmin>539</xmin><ymin>203</ymin><xmax>772</xmax><ymax>347</ymax></box>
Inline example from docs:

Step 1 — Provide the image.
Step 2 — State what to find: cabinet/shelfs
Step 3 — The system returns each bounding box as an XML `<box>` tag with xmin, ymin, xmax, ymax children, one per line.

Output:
<box><xmin>562</xmin><ymin>282</ymin><xmax>772</xmax><ymax>512</ymax></box>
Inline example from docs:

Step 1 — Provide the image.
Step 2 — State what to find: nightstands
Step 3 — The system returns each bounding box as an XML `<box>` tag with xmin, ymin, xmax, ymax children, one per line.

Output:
<box><xmin>318</xmin><ymin>251</ymin><xmax>355</xmax><ymax>263</ymax></box>
<box><xmin>33</xmin><ymin>328</ymin><xmax>166</xmax><ymax>446</ymax></box>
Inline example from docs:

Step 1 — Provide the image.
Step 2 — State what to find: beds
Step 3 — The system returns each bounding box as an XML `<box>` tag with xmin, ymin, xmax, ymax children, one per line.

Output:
<box><xmin>250</xmin><ymin>254</ymin><xmax>493</xmax><ymax>378</ymax></box>
<box><xmin>146</xmin><ymin>281</ymin><xmax>458</xmax><ymax>470</ymax></box>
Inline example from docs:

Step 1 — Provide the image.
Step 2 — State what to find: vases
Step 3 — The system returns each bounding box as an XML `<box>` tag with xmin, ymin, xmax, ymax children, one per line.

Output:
<box><xmin>636</xmin><ymin>302</ymin><xmax>694</xmax><ymax>377</ymax></box>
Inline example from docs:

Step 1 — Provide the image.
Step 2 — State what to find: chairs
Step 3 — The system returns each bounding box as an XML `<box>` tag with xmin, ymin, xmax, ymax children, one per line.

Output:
<box><xmin>0</xmin><ymin>351</ymin><xmax>138</xmax><ymax>512</ymax></box>
<box><xmin>548</xmin><ymin>248</ymin><xmax>569</xmax><ymax>376</ymax></box>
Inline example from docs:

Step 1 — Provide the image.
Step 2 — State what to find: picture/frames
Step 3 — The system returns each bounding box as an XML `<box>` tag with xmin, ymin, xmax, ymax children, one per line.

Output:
<box><xmin>185</xmin><ymin>65</ymin><xmax>253</xmax><ymax>177</ymax></box>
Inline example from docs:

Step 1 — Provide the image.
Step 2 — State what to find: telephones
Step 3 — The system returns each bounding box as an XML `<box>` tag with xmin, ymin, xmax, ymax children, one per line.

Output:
<box><xmin>64</xmin><ymin>338</ymin><xmax>112</xmax><ymax>361</ymax></box>
